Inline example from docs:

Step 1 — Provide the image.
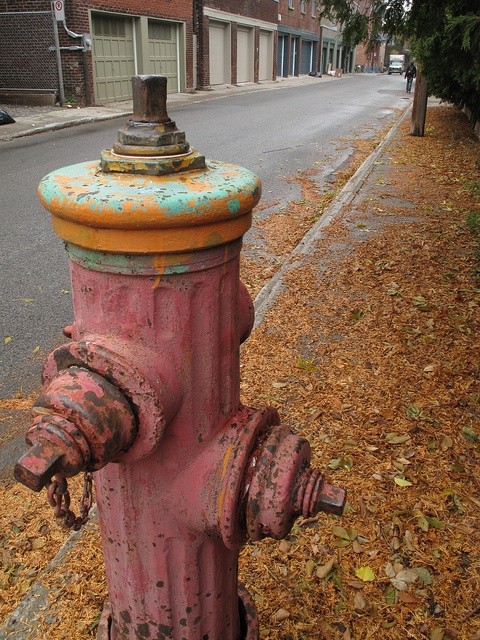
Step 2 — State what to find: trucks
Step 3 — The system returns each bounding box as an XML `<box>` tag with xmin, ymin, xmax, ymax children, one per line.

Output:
<box><xmin>388</xmin><ymin>55</ymin><xmax>405</xmax><ymax>75</ymax></box>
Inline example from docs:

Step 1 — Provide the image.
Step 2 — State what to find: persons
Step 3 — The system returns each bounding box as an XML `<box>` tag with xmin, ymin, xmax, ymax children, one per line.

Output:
<box><xmin>404</xmin><ymin>61</ymin><xmax>417</xmax><ymax>93</ymax></box>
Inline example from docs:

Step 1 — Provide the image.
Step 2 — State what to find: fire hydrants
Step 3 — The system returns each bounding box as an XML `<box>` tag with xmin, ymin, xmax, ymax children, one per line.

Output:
<box><xmin>15</xmin><ymin>76</ymin><xmax>346</xmax><ymax>640</ymax></box>
